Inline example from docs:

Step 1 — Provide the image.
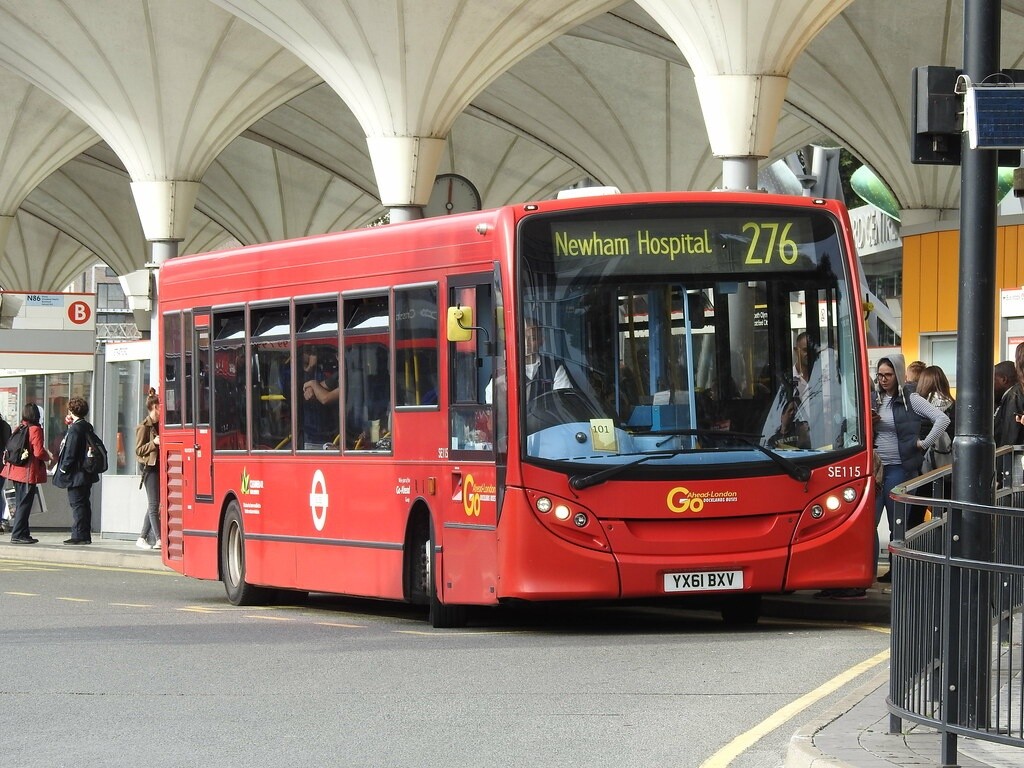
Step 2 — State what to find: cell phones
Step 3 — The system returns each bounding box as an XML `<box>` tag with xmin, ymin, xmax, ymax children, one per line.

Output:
<box><xmin>872</xmin><ymin>409</ymin><xmax>882</xmax><ymax>419</ymax></box>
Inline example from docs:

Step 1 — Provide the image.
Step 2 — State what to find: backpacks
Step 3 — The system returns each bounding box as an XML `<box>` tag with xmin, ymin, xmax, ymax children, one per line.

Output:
<box><xmin>73</xmin><ymin>424</ymin><xmax>108</xmax><ymax>473</ymax></box>
<box><xmin>921</xmin><ymin>433</ymin><xmax>953</xmax><ymax>474</ymax></box>
<box><xmin>6</xmin><ymin>422</ymin><xmax>41</xmax><ymax>466</ymax></box>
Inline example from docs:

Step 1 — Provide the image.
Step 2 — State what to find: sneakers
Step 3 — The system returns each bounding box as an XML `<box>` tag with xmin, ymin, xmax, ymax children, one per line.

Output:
<box><xmin>135</xmin><ymin>537</ymin><xmax>154</xmax><ymax>549</ymax></box>
<box><xmin>153</xmin><ymin>538</ymin><xmax>162</xmax><ymax>548</ymax></box>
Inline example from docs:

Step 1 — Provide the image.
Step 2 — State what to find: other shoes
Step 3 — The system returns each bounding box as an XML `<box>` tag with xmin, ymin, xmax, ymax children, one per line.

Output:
<box><xmin>833</xmin><ymin>588</ymin><xmax>868</xmax><ymax>599</ymax></box>
<box><xmin>877</xmin><ymin>569</ymin><xmax>892</xmax><ymax>582</ymax></box>
<box><xmin>64</xmin><ymin>538</ymin><xmax>92</xmax><ymax>545</ymax></box>
<box><xmin>11</xmin><ymin>536</ymin><xmax>39</xmax><ymax>544</ymax></box>
<box><xmin>813</xmin><ymin>589</ymin><xmax>838</xmax><ymax>599</ymax></box>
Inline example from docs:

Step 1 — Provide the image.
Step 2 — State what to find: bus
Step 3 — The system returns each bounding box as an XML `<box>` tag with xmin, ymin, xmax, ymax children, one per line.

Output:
<box><xmin>157</xmin><ymin>185</ymin><xmax>877</xmax><ymax>628</ymax></box>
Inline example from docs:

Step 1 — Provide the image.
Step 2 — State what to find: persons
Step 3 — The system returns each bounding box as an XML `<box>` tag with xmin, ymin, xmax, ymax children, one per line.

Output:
<box><xmin>1</xmin><ymin>402</ymin><xmax>52</xmax><ymax>544</ymax></box>
<box><xmin>877</xmin><ymin>366</ymin><xmax>955</xmax><ymax>583</ymax></box>
<box><xmin>1016</xmin><ymin>342</ymin><xmax>1024</xmax><ymax>426</ymax></box>
<box><xmin>137</xmin><ymin>388</ymin><xmax>163</xmax><ymax>550</ymax></box>
<box><xmin>52</xmin><ymin>396</ymin><xmax>100</xmax><ymax>545</ymax></box>
<box><xmin>792</xmin><ymin>330</ymin><xmax>809</xmax><ymax>433</ymax></box>
<box><xmin>905</xmin><ymin>361</ymin><xmax>926</xmax><ymax>384</ymax></box>
<box><xmin>302</xmin><ymin>346</ymin><xmax>340</xmax><ymax>450</ymax></box>
<box><xmin>345</xmin><ymin>367</ymin><xmax>391</xmax><ymax>450</ymax></box>
<box><xmin>484</xmin><ymin>318</ymin><xmax>574</xmax><ymax>410</ymax></box>
<box><xmin>0</xmin><ymin>415</ymin><xmax>11</xmax><ymax>533</ymax></box>
<box><xmin>871</xmin><ymin>353</ymin><xmax>951</xmax><ymax>583</ymax></box>
<box><xmin>994</xmin><ymin>361</ymin><xmax>1024</xmax><ymax>492</ymax></box>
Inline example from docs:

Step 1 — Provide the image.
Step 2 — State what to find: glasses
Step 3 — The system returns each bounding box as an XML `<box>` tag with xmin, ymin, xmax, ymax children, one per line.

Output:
<box><xmin>876</xmin><ymin>373</ymin><xmax>895</xmax><ymax>380</ymax></box>
